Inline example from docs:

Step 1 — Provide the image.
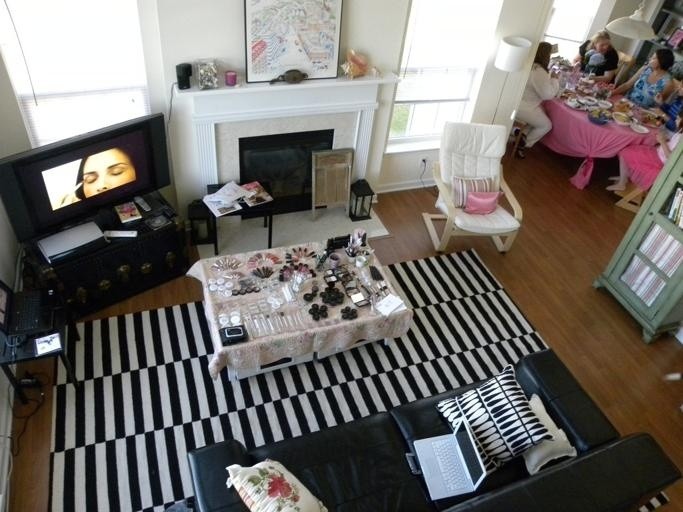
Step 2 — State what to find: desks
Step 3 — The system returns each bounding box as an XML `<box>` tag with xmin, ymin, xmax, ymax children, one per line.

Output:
<box><xmin>208</xmin><ymin>181</ymin><xmax>274</xmax><ymax>255</ymax></box>
<box><xmin>1</xmin><ymin>310</ymin><xmax>80</xmax><ymax>405</ymax></box>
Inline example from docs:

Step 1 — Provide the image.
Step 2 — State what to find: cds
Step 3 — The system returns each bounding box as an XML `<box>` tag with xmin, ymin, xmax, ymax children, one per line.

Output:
<box><xmin>149</xmin><ymin>215</ymin><xmax>166</xmax><ymax>227</ymax></box>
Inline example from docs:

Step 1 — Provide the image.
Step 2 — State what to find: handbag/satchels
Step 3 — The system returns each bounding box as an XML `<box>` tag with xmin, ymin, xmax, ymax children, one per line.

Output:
<box><xmin>271</xmin><ymin>70</ymin><xmax>308</xmax><ymax>83</ymax></box>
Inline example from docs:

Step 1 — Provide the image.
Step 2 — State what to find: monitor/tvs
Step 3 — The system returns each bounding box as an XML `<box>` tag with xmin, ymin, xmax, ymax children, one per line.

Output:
<box><xmin>0</xmin><ymin>112</ymin><xmax>172</xmax><ymax>242</ymax></box>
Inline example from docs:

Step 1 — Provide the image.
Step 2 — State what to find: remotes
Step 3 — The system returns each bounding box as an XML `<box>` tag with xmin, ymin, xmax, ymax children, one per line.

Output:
<box><xmin>134</xmin><ymin>196</ymin><xmax>151</xmax><ymax>212</ymax></box>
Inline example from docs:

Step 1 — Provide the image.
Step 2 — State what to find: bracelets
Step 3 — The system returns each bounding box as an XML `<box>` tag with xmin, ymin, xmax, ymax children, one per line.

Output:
<box><xmin>661</xmin><ymin>113</ymin><xmax>666</xmax><ymax>118</ymax></box>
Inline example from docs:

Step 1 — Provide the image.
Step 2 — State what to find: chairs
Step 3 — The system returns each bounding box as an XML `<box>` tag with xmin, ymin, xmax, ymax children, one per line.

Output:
<box><xmin>421</xmin><ymin>120</ymin><xmax>524</xmax><ymax>253</ymax></box>
<box><xmin>509</xmin><ymin>51</ymin><xmax>683</xmax><ymax>213</ymax></box>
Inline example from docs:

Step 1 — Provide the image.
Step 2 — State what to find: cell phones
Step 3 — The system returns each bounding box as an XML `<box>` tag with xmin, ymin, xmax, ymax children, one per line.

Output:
<box><xmin>405</xmin><ymin>452</ymin><xmax>421</xmax><ymax>475</ymax></box>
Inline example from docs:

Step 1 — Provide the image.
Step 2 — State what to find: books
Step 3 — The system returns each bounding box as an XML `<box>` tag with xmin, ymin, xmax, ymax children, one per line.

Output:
<box><xmin>239</xmin><ymin>181</ymin><xmax>274</xmax><ymax>208</ymax></box>
<box><xmin>144</xmin><ymin>215</ymin><xmax>172</xmax><ymax>232</ymax></box>
<box><xmin>661</xmin><ymin>187</ymin><xmax>683</xmax><ymax>231</ymax></box>
<box><xmin>113</xmin><ymin>202</ymin><xmax>143</xmax><ymax>224</ymax></box>
<box><xmin>201</xmin><ymin>195</ymin><xmax>242</xmax><ymax>219</ymax></box>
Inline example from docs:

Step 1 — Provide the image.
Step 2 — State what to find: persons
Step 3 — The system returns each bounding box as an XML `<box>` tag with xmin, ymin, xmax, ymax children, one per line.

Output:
<box><xmin>71</xmin><ymin>146</ymin><xmax>137</xmax><ymax>199</ymax></box>
<box><xmin>607</xmin><ymin>49</ymin><xmax>674</xmax><ymax>112</ymax></box>
<box><xmin>604</xmin><ymin>110</ymin><xmax>683</xmax><ymax>192</ymax></box>
<box><xmin>511</xmin><ymin>41</ymin><xmax>561</xmax><ymax>160</ymax></box>
<box><xmin>572</xmin><ymin>31</ymin><xmax>618</xmax><ymax>85</ymax></box>
<box><xmin>647</xmin><ymin>75</ymin><xmax>683</xmax><ymax>133</ymax></box>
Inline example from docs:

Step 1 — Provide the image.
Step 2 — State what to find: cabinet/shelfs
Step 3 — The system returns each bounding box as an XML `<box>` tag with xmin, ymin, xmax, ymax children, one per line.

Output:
<box><xmin>25</xmin><ymin>193</ymin><xmax>185</xmax><ymax>318</ymax></box>
<box><xmin>591</xmin><ymin>133</ymin><xmax>682</xmax><ymax>345</ymax></box>
<box><xmin>624</xmin><ymin>1</ymin><xmax>683</xmax><ymax>123</ymax></box>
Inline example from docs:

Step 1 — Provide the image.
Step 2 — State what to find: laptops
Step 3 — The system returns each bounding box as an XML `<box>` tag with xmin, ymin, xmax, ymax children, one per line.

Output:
<box><xmin>414</xmin><ymin>415</ymin><xmax>488</xmax><ymax>502</ymax></box>
<box><xmin>0</xmin><ymin>278</ymin><xmax>54</xmax><ymax>337</ymax></box>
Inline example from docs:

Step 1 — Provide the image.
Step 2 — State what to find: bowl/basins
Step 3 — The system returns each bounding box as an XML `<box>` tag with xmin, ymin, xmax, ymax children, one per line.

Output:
<box><xmin>583</xmin><ymin>95</ymin><xmax>612</xmax><ymax>110</ymax></box>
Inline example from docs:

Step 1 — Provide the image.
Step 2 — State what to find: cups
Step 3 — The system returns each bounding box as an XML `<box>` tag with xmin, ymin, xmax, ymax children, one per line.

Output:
<box><xmin>329</xmin><ymin>253</ymin><xmax>340</xmax><ymax>268</ymax></box>
<box><xmin>356</xmin><ymin>256</ymin><xmax>366</xmax><ymax>268</ymax></box>
<box><xmin>225</xmin><ymin>71</ymin><xmax>237</xmax><ymax>86</ymax></box>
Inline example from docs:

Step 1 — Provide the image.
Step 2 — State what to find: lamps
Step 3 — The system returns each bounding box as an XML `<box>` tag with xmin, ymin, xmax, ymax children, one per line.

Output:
<box><xmin>605</xmin><ymin>1</ymin><xmax>655</xmax><ymax>40</ymax></box>
<box><xmin>491</xmin><ymin>35</ymin><xmax>534</xmax><ymax>122</ymax></box>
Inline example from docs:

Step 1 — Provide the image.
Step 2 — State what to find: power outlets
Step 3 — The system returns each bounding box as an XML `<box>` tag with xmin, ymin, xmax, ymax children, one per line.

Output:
<box><xmin>419</xmin><ymin>158</ymin><xmax>427</xmax><ymax>168</ymax></box>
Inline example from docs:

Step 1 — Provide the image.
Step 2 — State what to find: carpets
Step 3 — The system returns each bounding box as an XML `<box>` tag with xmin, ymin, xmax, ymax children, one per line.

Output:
<box><xmin>49</xmin><ymin>249</ymin><xmax>673</xmax><ymax>512</ymax></box>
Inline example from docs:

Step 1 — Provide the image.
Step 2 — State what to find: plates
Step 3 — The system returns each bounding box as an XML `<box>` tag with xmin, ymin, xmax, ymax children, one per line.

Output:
<box><xmin>611</xmin><ymin>98</ymin><xmax>666</xmax><ymax>134</ymax></box>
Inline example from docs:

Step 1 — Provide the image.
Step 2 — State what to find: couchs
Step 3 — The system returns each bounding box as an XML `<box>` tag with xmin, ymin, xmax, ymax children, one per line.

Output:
<box><xmin>186</xmin><ymin>348</ymin><xmax>682</xmax><ymax>511</ymax></box>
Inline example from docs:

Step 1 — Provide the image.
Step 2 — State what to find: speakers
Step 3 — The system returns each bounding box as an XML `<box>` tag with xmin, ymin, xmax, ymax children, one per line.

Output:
<box><xmin>175</xmin><ymin>62</ymin><xmax>193</xmax><ymax>90</ymax></box>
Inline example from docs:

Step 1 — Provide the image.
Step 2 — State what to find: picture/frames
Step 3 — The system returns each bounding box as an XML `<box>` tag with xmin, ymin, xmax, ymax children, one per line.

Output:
<box><xmin>666</xmin><ymin>28</ymin><xmax>683</xmax><ymax>48</ymax></box>
<box><xmin>244</xmin><ymin>1</ymin><xmax>342</xmax><ymax>83</ymax></box>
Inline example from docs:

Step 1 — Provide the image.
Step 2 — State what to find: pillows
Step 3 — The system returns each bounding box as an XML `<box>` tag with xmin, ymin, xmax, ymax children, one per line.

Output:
<box><xmin>453</xmin><ymin>175</ymin><xmax>494</xmax><ymax>207</ymax></box>
<box><xmin>226</xmin><ymin>459</ymin><xmax>329</xmax><ymax>512</ymax></box>
<box><xmin>435</xmin><ymin>364</ymin><xmax>555</xmax><ymax>473</ymax></box>
<box><xmin>464</xmin><ymin>192</ymin><xmax>503</xmax><ymax>213</ymax></box>
<box><xmin>521</xmin><ymin>393</ymin><xmax>577</xmax><ymax>475</ymax></box>
